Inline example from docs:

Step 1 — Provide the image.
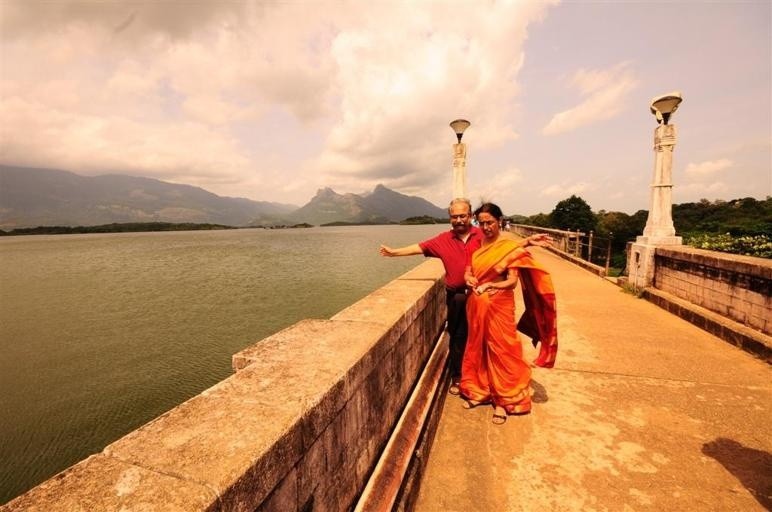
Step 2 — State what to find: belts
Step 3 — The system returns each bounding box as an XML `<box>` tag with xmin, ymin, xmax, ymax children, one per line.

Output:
<box><xmin>445</xmin><ymin>287</ymin><xmax>472</xmax><ymax>296</ymax></box>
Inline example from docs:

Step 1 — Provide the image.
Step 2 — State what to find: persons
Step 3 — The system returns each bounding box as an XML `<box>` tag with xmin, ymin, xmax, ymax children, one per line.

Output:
<box><xmin>455</xmin><ymin>200</ymin><xmax>561</xmax><ymax>427</ymax></box>
<box><xmin>471</xmin><ymin>213</ymin><xmax>479</xmax><ymax>226</ymax></box>
<box><xmin>504</xmin><ymin>219</ymin><xmax>510</xmax><ymax>232</ymax></box>
<box><xmin>501</xmin><ymin>218</ymin><xmax>506</xmax><ymax>232</ymax></box>
<box><xmin>378</xmin><ymin>197</ymin><xmax>558</xmax><ymax>396</ymax></box>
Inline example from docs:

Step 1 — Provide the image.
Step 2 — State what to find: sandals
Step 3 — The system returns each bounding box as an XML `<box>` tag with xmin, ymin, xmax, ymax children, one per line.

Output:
<box><xmin>449</xmin><ymin>383</ymin><xmax>460</xmax><ymax>395</ymax></box>
<box><xmin>462</xmin><ymin>400</ymin><xmax>474</xmax><ymax>409</ymax></box>
<box><xmin>492</xmin><ymin>414</ymin><xmax>507</xmax><ymax>425</ymax></box>
